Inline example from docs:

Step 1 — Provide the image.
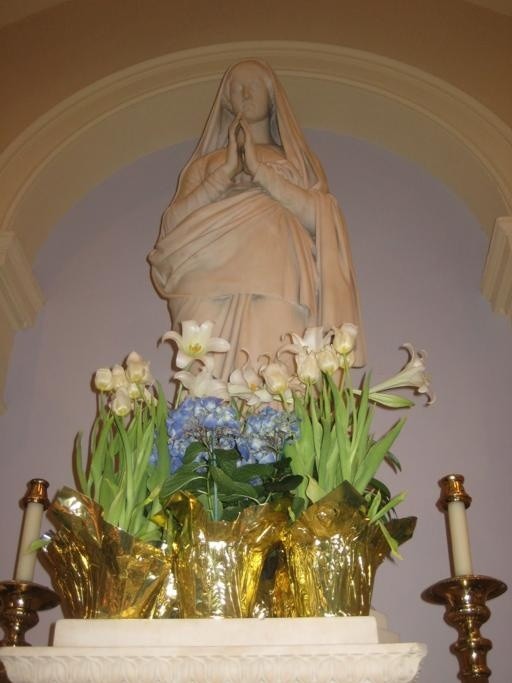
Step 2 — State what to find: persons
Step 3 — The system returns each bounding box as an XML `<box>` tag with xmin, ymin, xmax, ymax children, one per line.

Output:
<box><xmin>146</xmin><ymin>56</ymin><xmax>368</xmax><ymax>411</ymax></box>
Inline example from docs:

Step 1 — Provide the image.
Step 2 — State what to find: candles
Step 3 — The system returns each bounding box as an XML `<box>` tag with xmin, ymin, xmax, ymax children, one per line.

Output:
<box><xmin>14</xmin><ymin>501</ymin><xmax>43</xmax><ymax>584</ymax></box>
<box><xmin>445</xmin><ymin>501</ymin><xmax>472</xmax><ymax>577</ymax></box>
